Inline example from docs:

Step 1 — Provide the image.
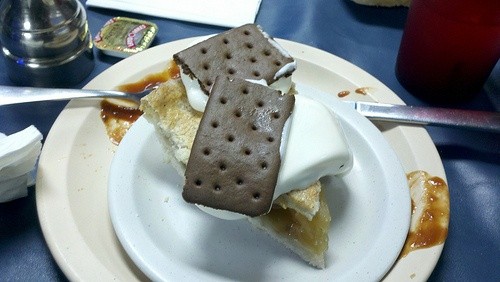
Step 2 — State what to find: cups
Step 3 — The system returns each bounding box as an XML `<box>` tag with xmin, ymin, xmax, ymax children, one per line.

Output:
<box><xmin>395</xmin><ymin>0</ymin><xmax>500</xmax><ymax>109</ymax></box>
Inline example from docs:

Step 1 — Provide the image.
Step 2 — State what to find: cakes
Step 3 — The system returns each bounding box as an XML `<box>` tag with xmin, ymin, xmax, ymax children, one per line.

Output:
<box><xmin>139</xmin><ymin>22</ymin><xmax>352</xmax><ymax>272</ymax></box>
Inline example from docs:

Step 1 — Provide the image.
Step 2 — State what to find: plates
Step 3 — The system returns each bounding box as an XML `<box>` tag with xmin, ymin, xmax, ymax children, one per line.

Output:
<box><xmin>107</xmin><ymin>81</ymin><xmax>411</xmax><ymax>282</ymax></box>
<box><xmin>35</xmin><ymin>31</ymin><xmax>450</xmax><ymax>282</ymax></box>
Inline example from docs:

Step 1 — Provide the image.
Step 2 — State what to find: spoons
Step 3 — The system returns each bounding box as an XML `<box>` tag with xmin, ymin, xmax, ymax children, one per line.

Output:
<box><xmin>0</xmin><ymin>86</ymin><xmax>160</xmax><ymax>105</ymax></box>
